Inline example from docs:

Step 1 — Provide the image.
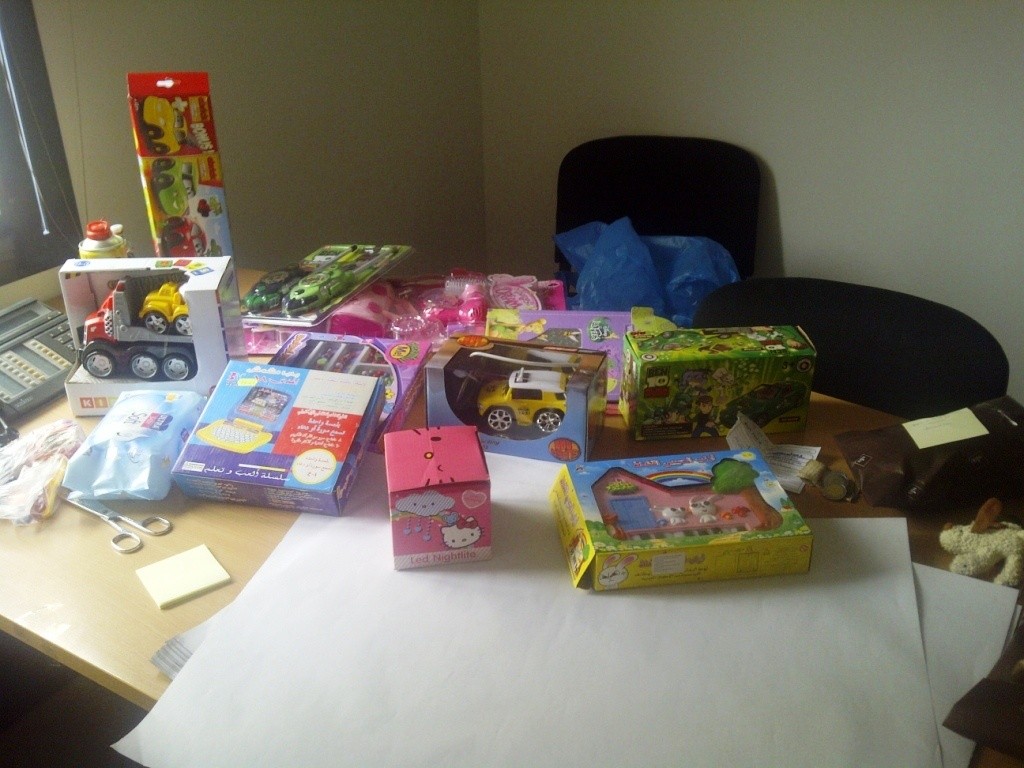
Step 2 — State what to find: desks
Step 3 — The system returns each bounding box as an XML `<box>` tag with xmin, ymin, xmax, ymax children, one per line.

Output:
<box><xmin>0</xmin><ymin>266</ymin><xmax>1024</xmax><ymax>768</ymax></box>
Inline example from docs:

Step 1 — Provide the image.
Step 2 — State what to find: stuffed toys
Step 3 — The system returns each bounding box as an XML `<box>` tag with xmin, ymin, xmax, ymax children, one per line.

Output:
<box><xmin>940</xmin><ymin>497</ymin><xmax>1024</xmax><ymax>587</ymax></box>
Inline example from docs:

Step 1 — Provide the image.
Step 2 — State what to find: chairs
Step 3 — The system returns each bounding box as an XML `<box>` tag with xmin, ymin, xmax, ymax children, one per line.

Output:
<box><xmin>690</xmin><ymin>276</ymin><xmax>1011</xmax><ymax>421</ymax></box>
<box><xmin>553</xmin><ymin>132</ymin><xmax>767</xmax><ymax>276</ymax></box>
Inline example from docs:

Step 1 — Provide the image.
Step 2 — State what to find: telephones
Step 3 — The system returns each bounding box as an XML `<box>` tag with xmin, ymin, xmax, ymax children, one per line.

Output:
<box><xmin>0</xmin><ymin>295</ymin><xmax>92</xmax><ymax>423</ymax></box>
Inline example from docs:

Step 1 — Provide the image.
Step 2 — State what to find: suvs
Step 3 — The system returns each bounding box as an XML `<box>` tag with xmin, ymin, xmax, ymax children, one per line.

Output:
<box><xmin>478</xmin><ymin>367</ymin><xmax>571</xmax><ymax>436</ymax></box>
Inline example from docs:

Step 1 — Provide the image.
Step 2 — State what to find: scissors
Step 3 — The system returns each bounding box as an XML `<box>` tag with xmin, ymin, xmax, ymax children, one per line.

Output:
<box><xmin>58</xmin><ymin>485</ymin><xmax>173</xmax><ymax>554</ymax></box>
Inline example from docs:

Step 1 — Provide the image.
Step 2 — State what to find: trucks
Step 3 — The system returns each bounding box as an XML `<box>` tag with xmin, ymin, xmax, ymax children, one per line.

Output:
<box><xmin>139</xmin><ymin>281</ymin><xmax>192</xmax><ymax>337</ymax></box>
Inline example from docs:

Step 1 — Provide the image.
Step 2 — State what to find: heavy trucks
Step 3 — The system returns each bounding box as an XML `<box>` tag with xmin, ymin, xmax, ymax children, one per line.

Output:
<box><xmin>81</xmin><ymin>276</ymin><xmax>197</xmax><ymax>382</ymax></box>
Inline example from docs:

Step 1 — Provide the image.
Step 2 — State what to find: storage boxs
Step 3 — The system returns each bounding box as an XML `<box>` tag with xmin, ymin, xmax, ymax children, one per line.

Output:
<box><xmin>381</xmin><ymin>422</ymin><xmax>493</xmax><ymax>573</ymax></box>
<box><xmin>549</xmin><ymin>448</ymin><xmax>813</xmax><ymax>593</ymax></box>
<box><xmin>617</xmin><ymin>324</ymin><xmax>818</xmax><ymax>441</ymax></box>
<box><xmin>267</xmin><ymin>332</ymin><xmax>438</xmax><ymax>453</ymax></box>
<box><xmin>422</xmin><ymin>334</ymin><xmax>611</xmax><ymax>463</ymax></box>
<box><xmin>171</xmin><ymin>360</ymin><xmax>386</xmax><ymax>515</ymax></box>
<box><xmin>126</xmin><ymin>70</ymin><xmax>234</xmax><ymax>257</ymax></box>
